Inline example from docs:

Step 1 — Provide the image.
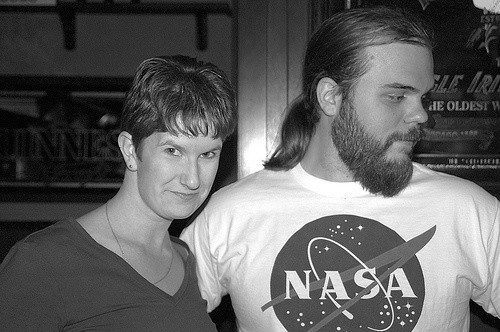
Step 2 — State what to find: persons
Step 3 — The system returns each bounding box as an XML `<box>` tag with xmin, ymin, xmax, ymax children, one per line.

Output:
<box><xmin>176</xmin><ymin>9</ymin><xmax>500</xmax><ymax>330</ymax></box>
<box><xmin>1</xmin><ymin>52</ymin><xmax>239</xmax><ymax>331</ymax></box>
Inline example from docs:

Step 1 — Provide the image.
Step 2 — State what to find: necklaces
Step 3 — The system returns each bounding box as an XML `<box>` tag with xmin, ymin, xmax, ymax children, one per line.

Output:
<box><xmin>105</xmin><ymin>198</ymin><xmax>174</xmax><ymax>285</ymax></box>
